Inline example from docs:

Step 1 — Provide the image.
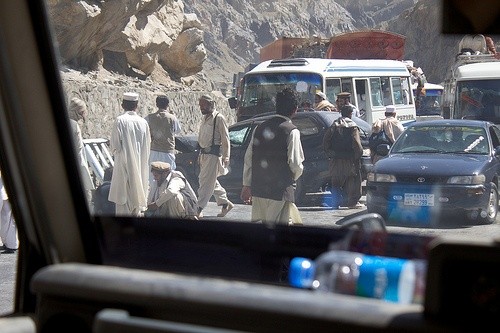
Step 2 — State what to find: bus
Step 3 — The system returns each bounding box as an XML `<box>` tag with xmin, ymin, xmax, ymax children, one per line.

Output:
<box><xmin>441</xmin><ymin>54</ymin><xmax>500</xmax><ymax>129</ymax></box>
<box><xmin>227</xmin><ymin>57</ymin><xmax>417</xmax><ymax>136</ymax></box>
<box><xmin>410</xmin><ymin>82</ymin><xmax>445</xmax><ymax>117</ymax></box>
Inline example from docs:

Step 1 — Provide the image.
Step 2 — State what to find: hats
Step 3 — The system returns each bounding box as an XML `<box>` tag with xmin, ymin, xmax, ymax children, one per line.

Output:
<box><xmin>340</xmin><ymin>106</ymin><xmax>353</xmax><ymax>112</ymax></box>
<box><xmin>386</xmin><ymin>108</ymin><xmax>396</xmax><ymax>112</ymax></box>
<box><xmin>316</xmin><ymin>92</ymin><xmax>326</xmax><ymax>100</ymax></box>
<box><xmin>301</xmin><ymin>99</ymin><xmax>313</xmax><ymax>106</ymax></box>
<box><xmin>150</xmin><ymin>162</ymin><xmax>171</xmax><ymax>174</ymax></box>
<box><xmin>69</xmin><ymin>98</ymin><xmax>86</xmax><ymax>113</ymax></box>
<box><xmin>337</xmin><ymin>92</ymin><xmax>351</xmax><ymax>98</ymax></box>
<box><xmin>123</xmin><ymin>92</ymin><xmax>139</xmax><ymax>101</ymax></box>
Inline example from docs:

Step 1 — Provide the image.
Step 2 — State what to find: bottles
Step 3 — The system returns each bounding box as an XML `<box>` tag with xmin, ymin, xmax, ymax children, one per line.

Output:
<box><xmin>288</xmin><ymin>249</ymin><xmax>427</xmax><ymax>309</ymax></box>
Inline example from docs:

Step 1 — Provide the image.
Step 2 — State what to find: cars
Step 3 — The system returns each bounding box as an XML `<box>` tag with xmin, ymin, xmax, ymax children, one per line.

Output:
<box><xmin>365</xmin><ymin>119</ymin><xmax>500</xmax><ymax>227</ymax></box>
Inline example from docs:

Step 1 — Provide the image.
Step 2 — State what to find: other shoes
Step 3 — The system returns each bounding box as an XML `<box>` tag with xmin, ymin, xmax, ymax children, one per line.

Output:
<box><xmin>0</xmin><ymin>244</ymin><xmax>7</xmax><ymax>250</ymax></box>
<box><xmin>1</xmin><ymin>249</ymin><xmax>15</xmax><ymax>254</ymax></box>
<box><xmin>350</xmin><ymin>202</ymin><xmax>365</xmax><ymax>209</ymax></box>
<box><xmin>217</xmin><ymin>200</ymin><xmax>234</xmax><ymax>217</ymax></box>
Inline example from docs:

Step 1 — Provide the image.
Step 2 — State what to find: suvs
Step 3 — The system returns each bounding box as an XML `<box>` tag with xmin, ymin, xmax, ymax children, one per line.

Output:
<box><xmin>173</xmin><ymin>108</ymin><xmax>374</xmax><ymax>209</ymax></box>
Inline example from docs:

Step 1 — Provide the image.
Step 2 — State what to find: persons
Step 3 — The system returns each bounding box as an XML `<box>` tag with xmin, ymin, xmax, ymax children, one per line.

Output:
<box><xmin>458</xmin><ymin>34</ymin><xmax>486</xmax><ymax>55</ymax></box>
<box><xmin>0</xmin><ymin>176</ymin><xmax>19</xmax><ymax>254</ymax></box>
<box><xmin>69</xmin><ymin>85</ymin><xmax>405</xmax><ymax>227</ymax></box>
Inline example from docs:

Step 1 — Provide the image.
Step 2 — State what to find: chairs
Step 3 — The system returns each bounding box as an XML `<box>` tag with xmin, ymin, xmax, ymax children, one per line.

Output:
<box><xmin>465</xmin><ymin>135</ymin><xmax>485</xmax><ymax>144</ymax></box>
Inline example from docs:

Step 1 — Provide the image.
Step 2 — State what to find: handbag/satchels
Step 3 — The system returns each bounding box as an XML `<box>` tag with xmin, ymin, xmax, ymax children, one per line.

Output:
<box><xmin>369</xmin><ymin>131</ymin><xmax>393</xmax><ymax>156</ymax></box>
